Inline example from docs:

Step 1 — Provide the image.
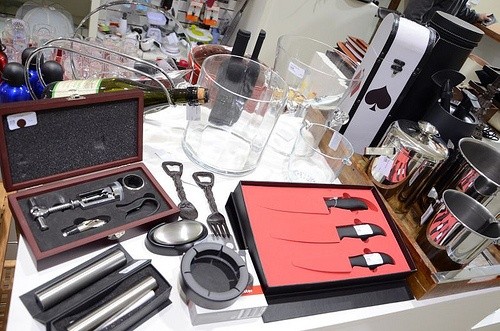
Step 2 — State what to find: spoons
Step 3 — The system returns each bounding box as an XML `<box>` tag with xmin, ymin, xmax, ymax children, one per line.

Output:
<box><xmin>162</xmin><ymin>161</ymin><xmax>198</xmax><ymax>221</ymax></box>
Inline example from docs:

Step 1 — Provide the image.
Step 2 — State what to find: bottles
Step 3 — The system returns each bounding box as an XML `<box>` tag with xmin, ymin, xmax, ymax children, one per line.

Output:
<box><xmin>43</xmin><ymin>78</ymin><xmax>209</xmax><ymax>112</ymax></box>
<box><xmin>119</xmin><ymin>13</ymin><xmax>127</xmax><ymax>33</ymax></box>
<box><xmin>208</xmin><ymin>29</ymin><xmax>252</xmax><ymax>124</ymax></box>
<box><xmin>241</xmin><ymin>30</ymin><xmax>266</xmax><ymax>110</ymax></box>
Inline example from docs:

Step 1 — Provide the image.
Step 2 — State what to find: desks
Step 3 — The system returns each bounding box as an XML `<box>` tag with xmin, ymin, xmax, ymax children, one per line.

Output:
<box><xmin>5</xmin><ymin>106</ymin><xmax>500</xmax><ymax>331</ymax></box>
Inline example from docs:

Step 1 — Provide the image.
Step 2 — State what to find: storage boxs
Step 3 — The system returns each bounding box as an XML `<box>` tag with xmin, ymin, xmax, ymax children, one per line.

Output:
<box><xmin>225</xmin><ymin>180</ymin><xmax>417</xmax><ymax>305</ymax></box>
<box><xmin>0</xmin><ymin>89</ymin><xmax>181</xmax><ymax>272</ymax></box>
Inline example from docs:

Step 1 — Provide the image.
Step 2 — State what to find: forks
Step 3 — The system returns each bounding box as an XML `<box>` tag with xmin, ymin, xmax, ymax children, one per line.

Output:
<box><xmin>193</xmin><ymin>172</ymin><xmax>232</xmax><ymax>238</ymax></box>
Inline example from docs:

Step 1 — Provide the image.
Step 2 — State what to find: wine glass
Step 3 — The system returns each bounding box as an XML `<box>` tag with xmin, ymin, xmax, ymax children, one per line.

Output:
<box><xmin>0</xmin><ymin>17</ymin><xmax>148</xmax><ymax>77</ymax></box>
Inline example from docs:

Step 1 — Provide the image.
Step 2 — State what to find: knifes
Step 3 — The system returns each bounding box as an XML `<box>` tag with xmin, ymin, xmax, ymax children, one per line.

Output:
<box><xmin>271</xmin><ymin>223</ymin><xmax>384</xmax><ymax>242</ymax></box>
<box><xmin>292</xmin><ymin>252</ymin><xmax>393</xmax><ymax>273</ymax></box>
<box><xmin>260</xmin><ymin>197</ymin><xmax>372</xmax><ymax>216</ymax></box>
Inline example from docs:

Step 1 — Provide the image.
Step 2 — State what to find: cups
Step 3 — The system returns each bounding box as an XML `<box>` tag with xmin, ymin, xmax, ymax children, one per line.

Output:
<box><xmin>181</xmin><ymin>54</ymin><xmax>289</xmax><ymax>174</ymax></box>
<box><xmin>414</xmin><ymin>189</ymin><xmax>500</xmax><ymax>269</ymax></box>
<box><xmin>414</xmin><ymin>137</ymin><xmax>500</xmax><ymax>234</ymax></box>
<box><xmin>288</xmin><ymin>122</ymin><xmax>354</xmax><ymax>184</ymax></box>
<box><xmin>264</xmin><ymin>35</ymin><xmax>365</xmax><ymax>156</ymax></box>
<box><xmin>363</xmin><ymin>119</ymin><xmax>448</xmax><ymax>214</ymax></box>
